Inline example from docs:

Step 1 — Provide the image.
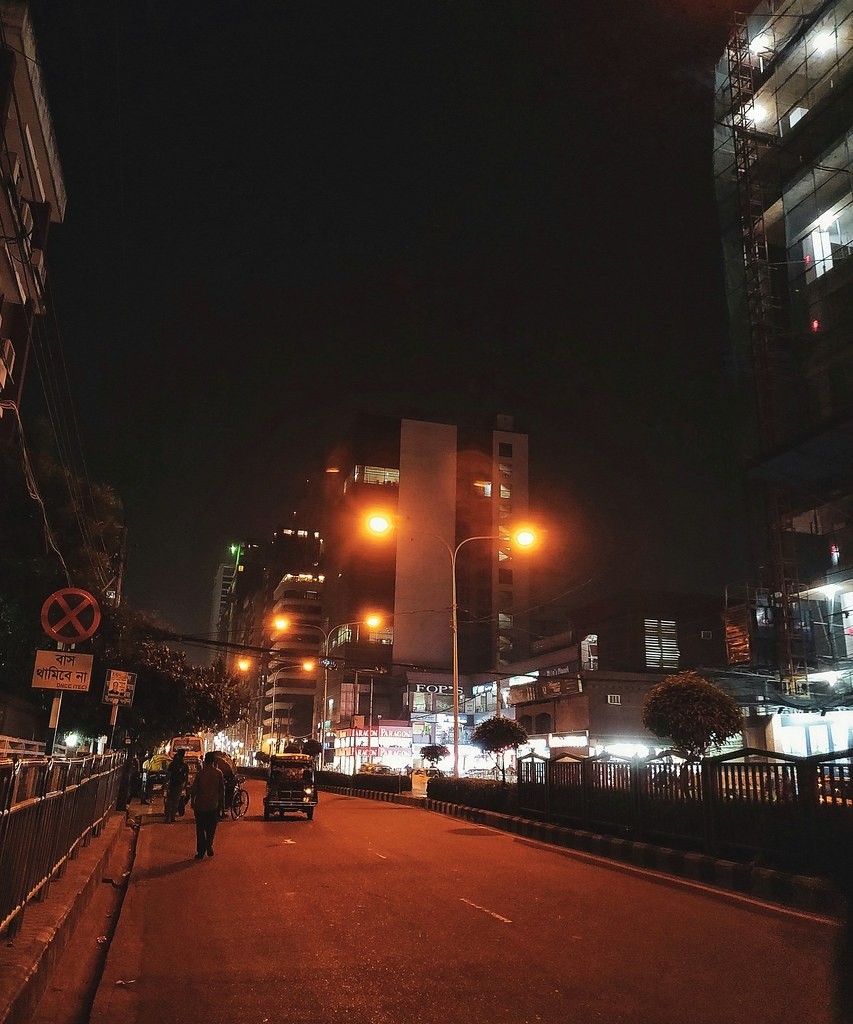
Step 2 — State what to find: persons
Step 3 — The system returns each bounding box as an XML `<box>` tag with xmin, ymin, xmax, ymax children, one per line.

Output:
<box><xmin>191</xmin><ymin>751</ymin><xmax>226</xmax><ymax>860</ymax></box>
<box><xmin>133</xmin><ymin>748</ymin><xmax>189</xmax><ymax>824</ymax></box>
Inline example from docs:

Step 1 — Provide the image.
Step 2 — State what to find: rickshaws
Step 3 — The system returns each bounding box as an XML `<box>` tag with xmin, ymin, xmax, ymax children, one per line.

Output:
<box><xmin>206</xmin><ymin>751</ymin><xmax>241</xmax><ymax>807</ymax></box>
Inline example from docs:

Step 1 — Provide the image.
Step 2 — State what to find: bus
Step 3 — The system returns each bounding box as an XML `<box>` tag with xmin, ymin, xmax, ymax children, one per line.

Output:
<box><xmin>170</xmin><ymin>736</ymin><xmax>205</xmax><ymax>764</ymax></box>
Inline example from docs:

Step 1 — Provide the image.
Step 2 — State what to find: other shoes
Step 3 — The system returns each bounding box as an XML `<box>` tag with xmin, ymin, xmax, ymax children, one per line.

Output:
<box><xmin>207</xmin><ymin>847</ymin><xmax>214</xmax><ymax>857</ymax></box>
<box><xmin>194</xmin><ymin>853</ymin><xmax>204</xmax><ymax>858</ymax></box>
<box><xmin>170</xmin><ymin>819</ymin><xmax>176</xmax><ymax>824</ymax></box>
<box><xmin>163</xmin><ymin>818</ymin><xmax>169</xmax><ymax>823</ymax></box>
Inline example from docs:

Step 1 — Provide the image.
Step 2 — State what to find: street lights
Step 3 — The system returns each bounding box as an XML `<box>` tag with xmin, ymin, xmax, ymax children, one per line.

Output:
<box><xmin>240</xmin><ymin>660</ymin><xmax>314</xmax><ymax>756</ymax></box>
<box><xmin>368</xmin><ymin>512</ymin><xmax>539</xmax><ymax>779</ymax></box>
<box><xmin>274</xmin><ymin>614</ymin><xmax>382</xmax><ymax>770</ymax></box>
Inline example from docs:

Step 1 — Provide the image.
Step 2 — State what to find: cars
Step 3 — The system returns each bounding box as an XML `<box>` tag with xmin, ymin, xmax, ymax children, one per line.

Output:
<box><xmin>263</xmin><ymin>752</ymin><xmax>319</xmax><ymax>821</ymax></box>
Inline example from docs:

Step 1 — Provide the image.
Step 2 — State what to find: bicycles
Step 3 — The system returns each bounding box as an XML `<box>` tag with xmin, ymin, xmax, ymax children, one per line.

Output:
<box><xmin>230</xmin><ymin>777</ymin><xmax>250</xmax><ymax>822</ymax></box>
